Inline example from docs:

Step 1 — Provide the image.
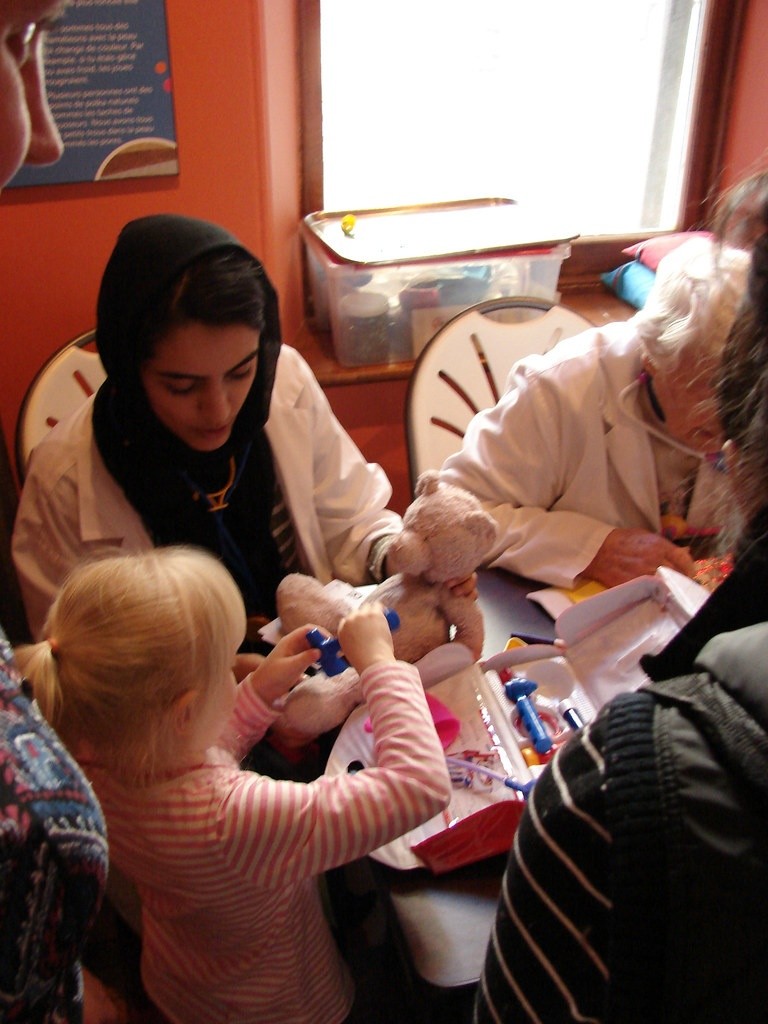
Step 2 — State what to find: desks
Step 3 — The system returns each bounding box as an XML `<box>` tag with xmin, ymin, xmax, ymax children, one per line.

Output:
<box><xmin>321</xmin><ymin>561</ymin><xmax>558</xmax><ymax>990</ymax></box>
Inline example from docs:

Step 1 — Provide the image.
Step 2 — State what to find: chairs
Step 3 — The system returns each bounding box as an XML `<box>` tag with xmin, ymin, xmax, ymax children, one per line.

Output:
<box><xmin>16</xmin><ymin>326</ymin><xmax>111</xmax><ymax>490</ymax></box>
<box><xmin>406</xmin><ymin>292</ymin><xmax>599</xmax><ymax>497</ymax></box>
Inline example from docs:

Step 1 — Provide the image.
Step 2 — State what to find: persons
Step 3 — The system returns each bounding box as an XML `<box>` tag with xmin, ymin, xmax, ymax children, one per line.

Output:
<box><xmin>8</xmin><ymin>543</ymin><xmax>451</xmax><ymax>1024</ymax></box>
<box><xmin>467</xmin><ymin>166</ymin><xmax>768</xmax><ymax>1023</ymax></box>
<box><xmin>0</xmin><ymin>0</ymin><xmax>69</xmax><ymax>197</ymax></box>
<box><xmin>11</xmin><ymin>213</ymin><xmax>479</xmax><ymax>689</ymax></box>
<box><xmin>440</xmin><ymin>235</ymin><xmax>752</xmax><ymax>588</ymax></box>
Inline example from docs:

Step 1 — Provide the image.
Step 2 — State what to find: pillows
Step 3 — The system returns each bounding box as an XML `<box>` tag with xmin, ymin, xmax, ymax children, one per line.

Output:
<box><xmin>597</xmin><ymin>259</ymin><xmax>658</xmax><ymax>311</ymax></box>
<box><xmin>621</xmin><ymin>230</ymin><xmax>716</xmax><ymax>273</ymax></box>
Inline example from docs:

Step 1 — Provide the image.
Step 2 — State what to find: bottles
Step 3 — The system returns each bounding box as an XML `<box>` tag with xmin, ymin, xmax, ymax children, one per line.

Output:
<box><xmin>341</xmin><ymin>293</ymin><xmax>391</xmax><ymax>364</ymax></box>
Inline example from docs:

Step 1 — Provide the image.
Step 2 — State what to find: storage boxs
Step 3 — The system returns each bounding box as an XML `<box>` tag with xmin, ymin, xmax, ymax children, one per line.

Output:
<box><xmin>301</xmin><ymin>192</ymin><xmax>576</xmax><ymax>372</ymax></box>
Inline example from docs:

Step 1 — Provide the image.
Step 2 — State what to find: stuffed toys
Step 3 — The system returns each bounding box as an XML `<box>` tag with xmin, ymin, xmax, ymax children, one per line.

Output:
<box><xmin>273</xmin><ymin>468</ymin><xmax>497</xmax><ymax>747</ymax></box>
